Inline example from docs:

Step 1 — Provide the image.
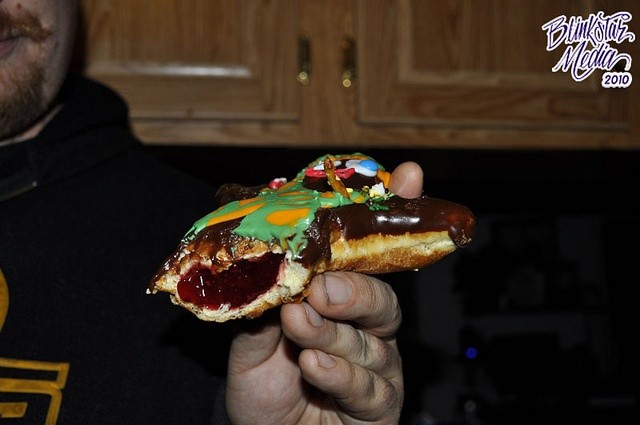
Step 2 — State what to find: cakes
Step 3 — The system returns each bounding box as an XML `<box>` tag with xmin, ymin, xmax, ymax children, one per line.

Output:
<box><xmin>142</xmin><ymin>152</ymin><xmax>478</xmax><ymax>324</ymax></box>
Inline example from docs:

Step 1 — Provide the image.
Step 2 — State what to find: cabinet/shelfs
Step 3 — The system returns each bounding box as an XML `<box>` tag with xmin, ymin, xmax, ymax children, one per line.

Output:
<box><xmin>78</xmin><ymin>0</ymin><xmax>640</xmax><ymax>151</ymax></box>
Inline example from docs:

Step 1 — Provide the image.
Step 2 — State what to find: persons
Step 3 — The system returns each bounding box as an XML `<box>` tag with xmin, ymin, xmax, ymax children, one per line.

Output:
<box><xmin>0</xmin><ymin>0</ymin><xmax>426</xmax><ymax>425</ymax></box>
